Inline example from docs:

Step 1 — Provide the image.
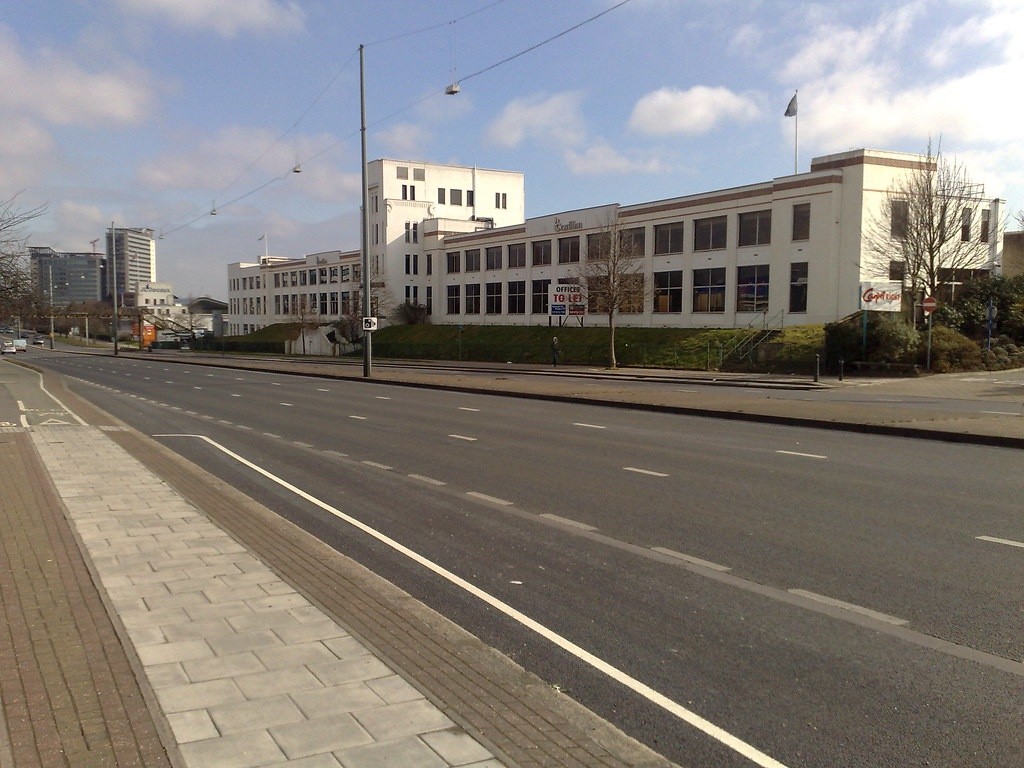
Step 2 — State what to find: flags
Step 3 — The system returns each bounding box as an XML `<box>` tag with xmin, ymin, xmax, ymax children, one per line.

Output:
<box><xmin>785</xmin><ymin>94</ymin><xmax>798</xmax><ymax>117</ymax></box>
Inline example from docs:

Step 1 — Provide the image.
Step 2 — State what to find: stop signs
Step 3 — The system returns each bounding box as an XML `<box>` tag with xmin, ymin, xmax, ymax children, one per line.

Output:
<box><xmin>923</xmin><ymin>298</ymin><xmax>937</xmax><ymax>312</ymax></box>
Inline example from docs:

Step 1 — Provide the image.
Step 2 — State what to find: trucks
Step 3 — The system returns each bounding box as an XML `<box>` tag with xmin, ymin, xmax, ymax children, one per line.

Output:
<box><xmin>13</xmin><ymin>340</ymin><xmax>26</xmax><ymax>352</ymax></box>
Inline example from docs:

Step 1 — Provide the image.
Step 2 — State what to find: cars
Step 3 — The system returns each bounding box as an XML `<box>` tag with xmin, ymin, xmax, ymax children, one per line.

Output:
<box><xmin>32</xmin><ymin>336</ymin><xmax>44</xmax><ymax>345</ymax></box>
<box><xmin>21</xmin><ymin>334</ymin><xmax>29</xmax><ymax>338</ymax></box>
<box><xmin>0</xmin><ymin>328</ymin><xmax>3</xmax><ymax>333</ymax></box>
<box><xmin>4</xmin><ymin>341</ymin><xmax>12</xmax><ymax>347</ymax></box>
<box><xmin>7</xmin><ymin>328</ymin><xmax>14</xmax><ymax>333</ymax></box>
<box><xmin>1</xmin><ymin>344</ymin><xmax>16</xmax><ymax>355</ymax></box>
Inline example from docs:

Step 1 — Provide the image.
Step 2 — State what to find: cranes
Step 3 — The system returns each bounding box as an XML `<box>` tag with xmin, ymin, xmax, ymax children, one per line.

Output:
<box><xmin>90</xmin><ymin>239</ymin><xmax>100</xmax><ymax>253</ymax></box>
